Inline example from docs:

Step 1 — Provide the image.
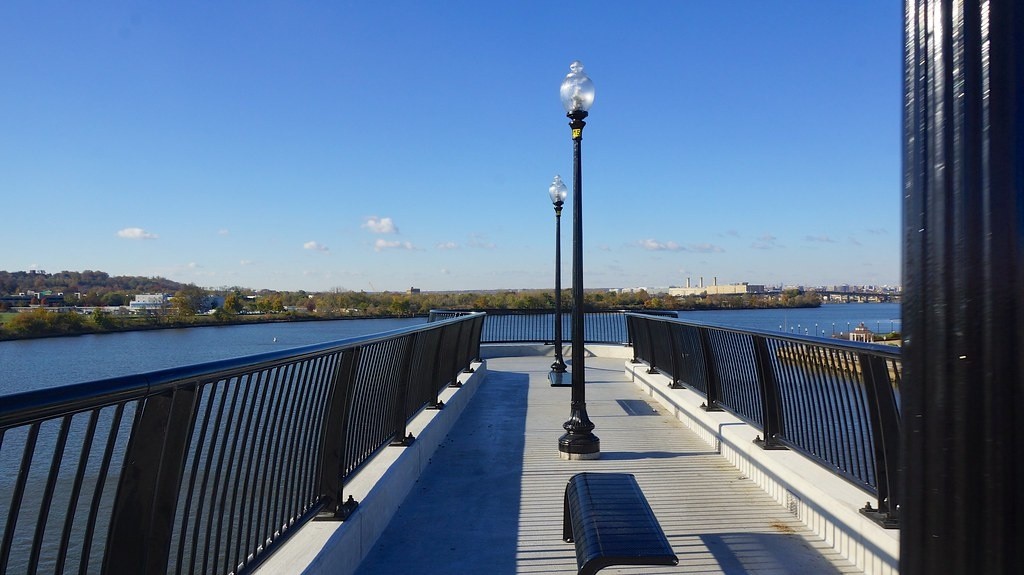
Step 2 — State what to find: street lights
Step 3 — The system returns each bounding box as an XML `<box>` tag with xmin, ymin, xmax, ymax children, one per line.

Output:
<box><xmin>548</xmin><ymin>177</ymin><xmax>567</xmax><ymax>373</ymax></box>
<box><xmin>558</xmin><ymin>60</ymin><xmax>601</xmax><ymax>463</ymax></box>
<box><xmin>779</xmin><ymin>319</ymin><xmax>894</xmax><ymax>361</ymax></box>
